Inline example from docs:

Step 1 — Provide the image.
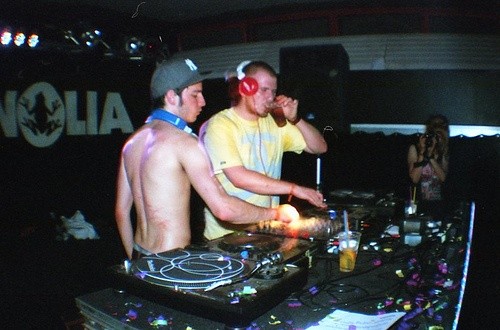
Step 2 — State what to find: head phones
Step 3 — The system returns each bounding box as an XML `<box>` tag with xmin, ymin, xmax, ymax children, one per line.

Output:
<box><xmin>236</xmin><ymin>61</ymin><xmax>258</xmax><ymax>96</ymax></box>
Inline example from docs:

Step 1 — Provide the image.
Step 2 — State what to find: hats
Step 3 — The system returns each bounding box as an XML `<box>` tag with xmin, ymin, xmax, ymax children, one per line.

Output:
<box><xmin>150</xmin><ymin>56</ymin><xmax>214</xmax><ymax>98</ymax></box>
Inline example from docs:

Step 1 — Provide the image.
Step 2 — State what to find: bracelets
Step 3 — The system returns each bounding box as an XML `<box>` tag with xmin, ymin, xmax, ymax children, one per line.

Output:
<box><xmin>288</xmin><ymin>183</ymin><xmax>296</xmax><ymax>202</ymax></box>
<box><xmin>288</xmin><ymin>115</ymin><xmax>301</xmax><ymax>125</ymax></box>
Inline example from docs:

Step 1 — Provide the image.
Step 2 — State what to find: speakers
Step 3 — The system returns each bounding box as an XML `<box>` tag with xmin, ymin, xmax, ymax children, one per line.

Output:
<box><xmin>278</xmin><ymin>44</ymin><xmax>351</xmax><ymax>143</ymax></box>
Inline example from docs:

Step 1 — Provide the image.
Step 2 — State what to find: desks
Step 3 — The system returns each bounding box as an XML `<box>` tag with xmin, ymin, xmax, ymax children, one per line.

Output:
<box><xmin>74</xmin><ymin>200</ymin><xmax>475</xmax><ymax>330</ymax></box>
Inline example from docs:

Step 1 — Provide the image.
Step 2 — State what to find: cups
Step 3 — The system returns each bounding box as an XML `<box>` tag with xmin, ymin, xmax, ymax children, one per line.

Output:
<box><xmin>264</xmin><ymin>99</ymin><xmax>287</xmax><ymax>127</ymax></box>
<box><xmin>338</xmin><ymin>231</ymin><xmax>361</xmax><ymax>272</ymax></box>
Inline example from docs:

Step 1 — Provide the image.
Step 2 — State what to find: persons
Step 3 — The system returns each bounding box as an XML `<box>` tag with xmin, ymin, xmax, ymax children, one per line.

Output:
<box><xmin>116</xmin><ymin>56</ymin><xmax>300</xmax><ymax>266</ymax></box>
<box><xmin>408</xmin><ymin>115</ymin><xmax>449</xmax><ymax>201</ymax></box>
<box><xmin>199</xmin><ymin>60</ymin><xmax>328</xmax><ymax>244</ymax></box>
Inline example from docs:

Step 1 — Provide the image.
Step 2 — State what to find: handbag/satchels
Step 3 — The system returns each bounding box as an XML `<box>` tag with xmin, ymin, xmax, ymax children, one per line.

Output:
<box><xmin>60</xmin><ymin>210</ymin><xmax>100</xmax><ymax>240</ymax></box>
